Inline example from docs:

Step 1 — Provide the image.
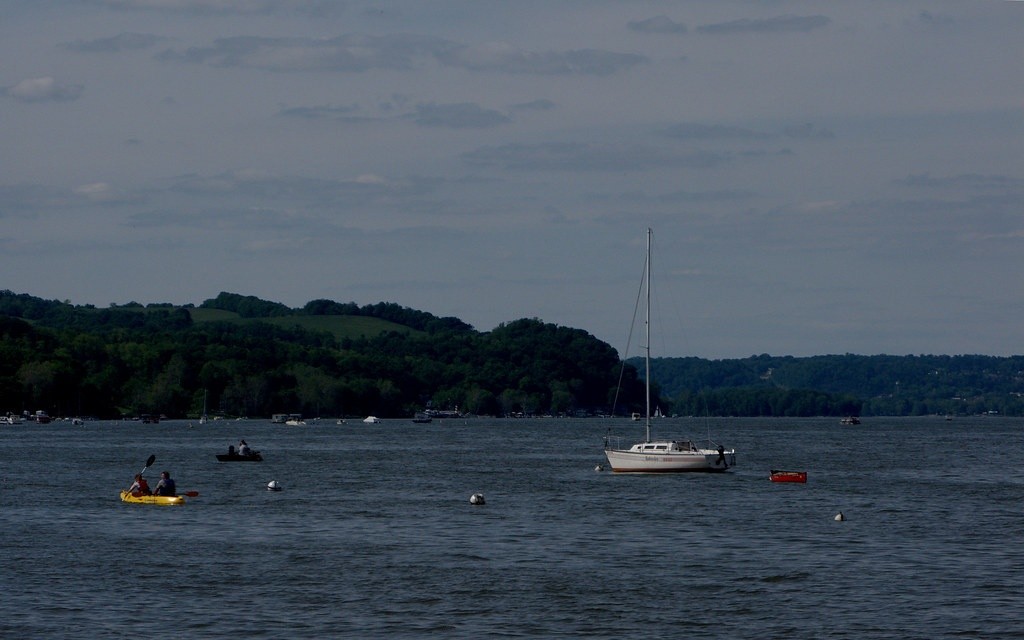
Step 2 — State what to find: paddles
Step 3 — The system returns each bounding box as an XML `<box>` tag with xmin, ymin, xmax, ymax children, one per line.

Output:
<box><xmin>133</xmin><ymin>490</ymin><xmax>199</xmax><ymax>497</ymax></box>
<box><xmin>122</xmin><ymin>455</ymin><xmax>156</xmax><ymax>501</ymax></box>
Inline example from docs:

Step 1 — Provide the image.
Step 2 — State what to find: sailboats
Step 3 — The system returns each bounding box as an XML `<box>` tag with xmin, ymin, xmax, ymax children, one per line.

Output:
<box><xmin>602</xmin><ymin>227</ymin><xmax>737</xmax><ymax>473</ymax></box>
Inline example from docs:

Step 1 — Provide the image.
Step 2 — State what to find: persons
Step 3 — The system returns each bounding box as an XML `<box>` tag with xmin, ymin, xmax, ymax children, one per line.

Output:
<box><xmin>122</xmin><ymin>472</ymin><xmax>153</xmax><ymax>498</ymax></box>
<box><xmin>238</xmin><ymin>439</ymin><xmax>251</xmax><ymax>457</ymax></box>
<box><xmin>152</xmin><ymin>470</ymin><xmax>177</xmax><ymax>497</ymax></box>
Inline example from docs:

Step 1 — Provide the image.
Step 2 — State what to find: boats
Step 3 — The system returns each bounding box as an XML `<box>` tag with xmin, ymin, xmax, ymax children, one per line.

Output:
<box><xmin>120</xmin><ymin>489</ymin><xmax>186</xmax><ymax>506</ymax></box>
<box><xmin>271</xmin><ymin>414</ymin><xmax>307</xmax><ymax>427</ymax></box>
<box><xmin>411</xmin><ymin>410</ymin><xmax>433</xmax><ymax>423</ymax></box>
<box><xmin>0</xmin><ymin>409</ymin><xmax>86</xmax><ymax>426</ymax></box>
<box><xmin>215</xmin><ymin>452</ymin><xmax>265</xmax><ymax>462</ymax></box>
<box><xmin>768</xmin><ymin>469</ymin><xmax>808</xmax><ymax>484</ymax></box>
